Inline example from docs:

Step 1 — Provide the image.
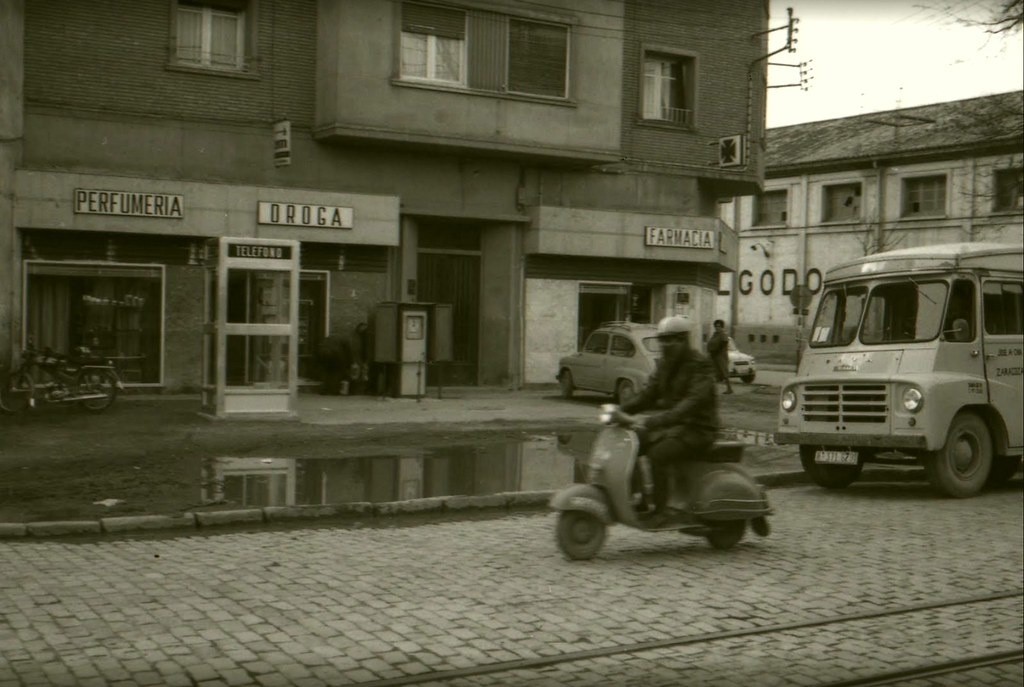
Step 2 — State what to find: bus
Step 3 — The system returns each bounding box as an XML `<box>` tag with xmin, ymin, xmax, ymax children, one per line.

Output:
<box><xmin>772</xmin><ymin>240</ymin><xmax>1023</xmax><ymax>497</ymax></box>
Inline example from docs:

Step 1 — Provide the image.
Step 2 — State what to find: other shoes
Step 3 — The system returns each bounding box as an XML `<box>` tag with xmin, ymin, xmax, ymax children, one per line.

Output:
<box><xmin>638</xmin><ymin>511</ymin><xmax>658</xmax><ymax>520</ymax></box>
<box><xmin>723</xmin><ymin>390</ymin><xmax>733</xmax><ymax>394</ymax></box>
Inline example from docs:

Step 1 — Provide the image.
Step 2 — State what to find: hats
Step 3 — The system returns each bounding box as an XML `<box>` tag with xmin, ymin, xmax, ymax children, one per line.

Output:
<box><xmin>657</xmin><ymin>317</ymin><xmax>689</xmax><ymax>337</ymax></box>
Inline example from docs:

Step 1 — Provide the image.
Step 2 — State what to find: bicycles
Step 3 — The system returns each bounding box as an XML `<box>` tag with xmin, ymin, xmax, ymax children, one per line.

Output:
<box><xmin>0</xmin><ymin>334</ymin><xmax>123</xmax><ymax>415</ymax></box>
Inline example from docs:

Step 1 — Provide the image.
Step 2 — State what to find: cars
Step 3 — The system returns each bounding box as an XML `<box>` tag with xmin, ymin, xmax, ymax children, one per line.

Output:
<box><xmin>557</xmin><ymin>323</ymin><xmax>668</xmax><ymax>403</ymax></box>
<box><xmin>702</xmin><ymin>333</ymin><xmax>756</xmax><ymax>384</ymax></box>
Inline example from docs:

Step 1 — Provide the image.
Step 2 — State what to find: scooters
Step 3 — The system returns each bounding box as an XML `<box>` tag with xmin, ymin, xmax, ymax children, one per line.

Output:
<box><xmin>550</xmin><ymin>404</ymin><xmax>771</xmax><ymax>562</ymax></box>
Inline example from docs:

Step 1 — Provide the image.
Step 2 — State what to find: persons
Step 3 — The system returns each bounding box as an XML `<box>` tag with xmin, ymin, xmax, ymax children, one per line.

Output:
<box><xmin>707</xmin><ymin>319</ymin><xmax>734</xmax><ymax>395</ymax></box>
<box><xmin>618</xmin><ymin>317</ymin><xmax>720</xmax><ymax>528</ymax></box>
